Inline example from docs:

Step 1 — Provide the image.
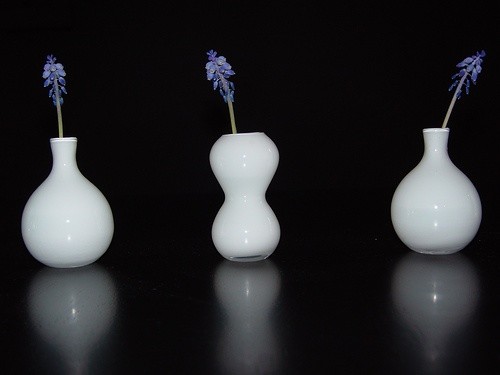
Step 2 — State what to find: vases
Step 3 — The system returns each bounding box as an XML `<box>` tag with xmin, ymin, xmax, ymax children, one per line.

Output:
<box><xmin>20</xmin><ymin>137</ymin><xmax>114</xmax><ymax>268</ymax></box>
<box><xmin>209</xmin><ymin>132</ymin><xmax>280</xmax><ymax>263</ymax></box>
<box><xmin>391</xmin><ymin>128</ymin><xmax>482</xmax><ymax>256</ymax></box>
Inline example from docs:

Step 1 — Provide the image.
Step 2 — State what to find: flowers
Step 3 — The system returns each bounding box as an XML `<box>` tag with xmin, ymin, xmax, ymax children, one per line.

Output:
<box><xmin>205</xmin><ymin>49</ymin><xmax>237</xmax><ymax>134</ymax></box>
<box><xmin>441</xmin><ymin>49</ymin><xmax>488</xmax><ymax>127</ymax></box>
<box><xmin>42</xmin><ymin>54</ymin><xmax>68</xmax><ymax>138</ymax></box>
